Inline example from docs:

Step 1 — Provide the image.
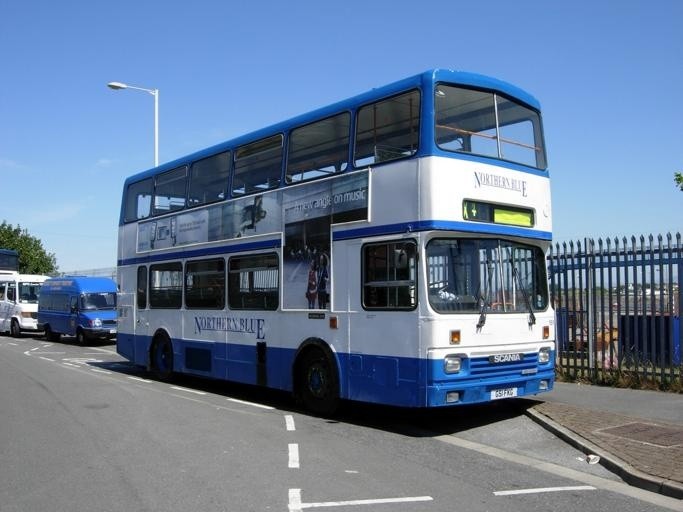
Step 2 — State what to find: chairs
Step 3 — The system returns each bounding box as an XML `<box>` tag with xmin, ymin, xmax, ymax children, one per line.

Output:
<box><xmin>152</xmin><ymin>143</ymin><xmax>416</xmax><ymax>219</ymax></box>
<box><xmin>164</xmin><ymin>286</ymin><xmax>277</xmax><ymax>309</ymax></box>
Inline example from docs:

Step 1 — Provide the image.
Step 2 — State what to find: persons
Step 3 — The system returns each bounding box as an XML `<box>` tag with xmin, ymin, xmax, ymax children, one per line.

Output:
<box><xmin>317</xmin><ymin>253</ymin><xmax>330</xmax><ymax>308</ymax></box>
<box><xmin>237</xmin><ymin>195</ymin><xmax>266</xmax><ymax>238</ymax></box>
<box><xmin>305</xmin><ymin>259</ymin><xmax>318</xmax><ymax>309</ymax></box>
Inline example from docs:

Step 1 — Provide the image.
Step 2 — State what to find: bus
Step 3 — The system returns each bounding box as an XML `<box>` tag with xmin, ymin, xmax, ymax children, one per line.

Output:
<box><xmin>116</xmin><ymin>67</ymin><xmax>569</xmax><ymax>413</ymax></box>
<box><xmin>0</xmin><ymin>249</ymin><xmax>19</xmax><ymax>287</ymax></box>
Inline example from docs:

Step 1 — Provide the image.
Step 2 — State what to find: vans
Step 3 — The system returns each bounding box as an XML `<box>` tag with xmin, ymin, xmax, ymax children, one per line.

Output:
<box><xmin>0</xmin><ymin>274</ymin><xmax>54</xmax><ymax>336</ymax></box>
<box><xmin>36</xmin><ymin>275</ymin><xmax>119</xmax><ymax>342</ymax></box>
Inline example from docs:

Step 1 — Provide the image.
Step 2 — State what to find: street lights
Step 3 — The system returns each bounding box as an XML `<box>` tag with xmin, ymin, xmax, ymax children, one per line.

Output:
<box><xmin>105</xmin><ymin>80</ymin><xmax>161</xmax><ymax>168</ymax></box>
